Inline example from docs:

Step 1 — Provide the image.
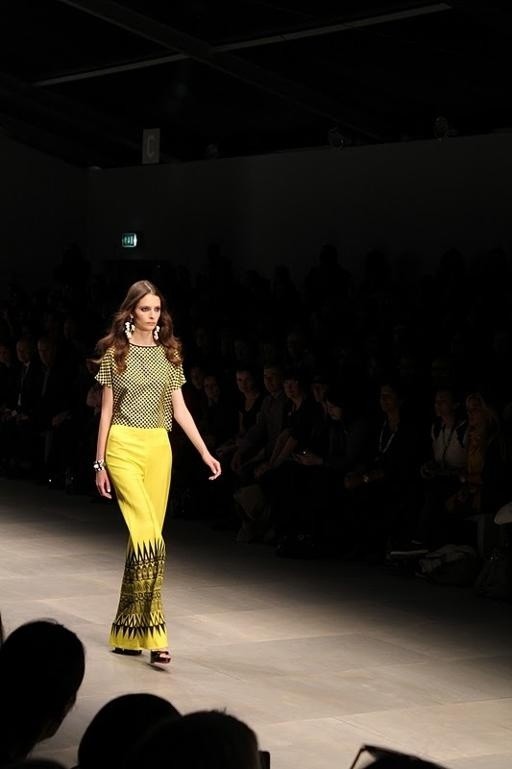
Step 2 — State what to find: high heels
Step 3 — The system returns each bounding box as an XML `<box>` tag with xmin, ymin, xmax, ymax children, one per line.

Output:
<box><xmin>149</xmin><ymin>649</ymin><xmax>172</xmax><ymax>664</ymax></box>
<box><xmin>115</xmin><ymin>646</ymin><xmax>142</xmax><ymax>655</ymax></box>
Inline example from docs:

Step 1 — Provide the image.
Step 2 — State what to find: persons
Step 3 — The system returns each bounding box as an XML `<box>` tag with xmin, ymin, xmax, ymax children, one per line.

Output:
<box><xmin>71</xmin><ymin>693</ymin><xmax>258</xmax><ymax>769</ymax></box>
<box><xmin>1</xmin><ymin>317</ymin><xmax>512</xmax><ymax>591</ymax></box>
<box><xmin>1</xmin><ymin>619</ymin><xmax>85</xmax><ymax>769</ymax></box>
<box><xmin>94</xmin><ymin>280</ymin><xmax>221</xmax><ymax>663</ymax></box>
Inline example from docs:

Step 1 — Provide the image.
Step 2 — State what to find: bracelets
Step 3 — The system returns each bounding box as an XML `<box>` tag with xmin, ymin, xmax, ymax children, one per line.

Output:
<box><xmin>95</xmin><ymin>460</ymin><xmax>104</xmax><ymax>472</ymax></box>
